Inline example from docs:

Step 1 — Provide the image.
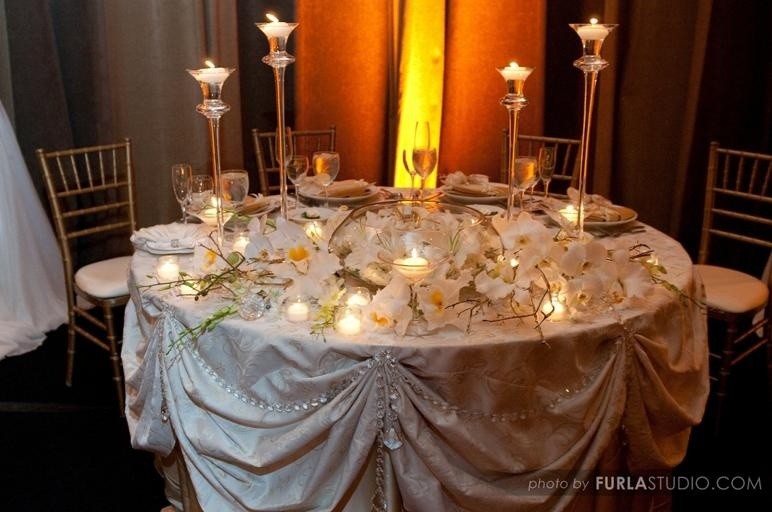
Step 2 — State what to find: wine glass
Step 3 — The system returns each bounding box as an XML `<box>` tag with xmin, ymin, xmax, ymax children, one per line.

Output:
<box><xmin>171</xmin><ymin>164</ymin><xmax>213</xmax><ymax>223</ymax></box>
<box><xmin>515</xmin><ymin>148</ymin><xmax>555</xmax><ymax>209</ymax></box>
<box><xmin>405</xmin><ymin>121</ymin><xmax>436</xmax><ymax>202</ymax></box>
<box><xmin>313</xmin><ymin>152</ymin><xmax>338</xmax><ymax>209</ymax></box>
<box><xmin>221</xmin><ymin>172</ymin><xmax>249</xmax><ymax>242</ymax></box>
<box><xmin>276</xmin><ymin>128</ymin><xmax>307</xmax><ymax>210</ymax></box>
<box><xmin>378</xmin><ymin>243</ymin><xmax>448</xmax><ymax>337</ymax></box>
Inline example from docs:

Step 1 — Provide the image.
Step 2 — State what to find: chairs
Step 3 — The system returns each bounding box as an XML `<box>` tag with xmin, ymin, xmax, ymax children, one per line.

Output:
<box><xmin>250</xmin><ymin>121</ymin><xmax>339</xmax><ymax>198</ymax></box>
<box><xmin>36</xmin><ymin>138</ymin><xmax>142</xmax><ymax>416</ymax></box>
<box><xmin>497</xmin><ymin>127</ymin><xmax>582</xmax><ymax>197</ymax></box>
<box><xmin>687</xmin><ymin>141</ymin><xmax>771</xmax><ymax>424</ymax></box>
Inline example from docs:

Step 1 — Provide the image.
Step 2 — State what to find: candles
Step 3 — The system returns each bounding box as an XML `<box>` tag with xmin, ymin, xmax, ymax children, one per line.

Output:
<box><xmin>262</xmin><ymin>12</ymin><xmax>290</xmax><ymax>38</ymax></box>
<box><xmin>198</xmin><ymin>57</ymin><xmax>228</xmax><ymax>83</ymax></box>
<box><xmin>577</xmin><ymin>17</ymin><xmax>609</xmax><ymax>40</ymax></box>
<box><xmin>502</xmin><ymin>61</ymin><xmax>528</xmax><ymax>80</ymax></box>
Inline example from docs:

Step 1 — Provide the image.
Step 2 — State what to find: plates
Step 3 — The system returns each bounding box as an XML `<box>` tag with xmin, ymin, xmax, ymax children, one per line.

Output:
<box><xmin>583</xmin><ymin>205</ymin><xmax>638</xmax><ymax>228</ymax></box>
<box><xmin>440</xmin><ymin>183</ymin><xmax>516</xmax><ymax>201</ymax></box>
<box><xmin>463</xmin><ymin>203</ymin><xmax>503</xmax><ymax>221</ymax></box>
<box><xmin>250</xmin><ymin>197</ymin><xmax>276</xmax><ymax>216</ymax></box>
<box><xmin>294</xmin><ymin>180</ymin><xmax>379</xmax><ymax>203</ymax></box>
<box><xmin>130</xmin><ymin>224</ymin><xmax>209</xmax><ymax>254</ymax></box>
<box><xmin>284</xmin><ymin>212</ymin><xmax>337</xmax><ymax>223</ymax></box>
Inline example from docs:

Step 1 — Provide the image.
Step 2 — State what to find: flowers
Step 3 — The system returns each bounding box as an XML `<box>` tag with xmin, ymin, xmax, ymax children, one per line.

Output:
<box><xmin>128</xmin><ymin>209</ymin><xmax>723</xmax><ymax>361</ymax></box>
